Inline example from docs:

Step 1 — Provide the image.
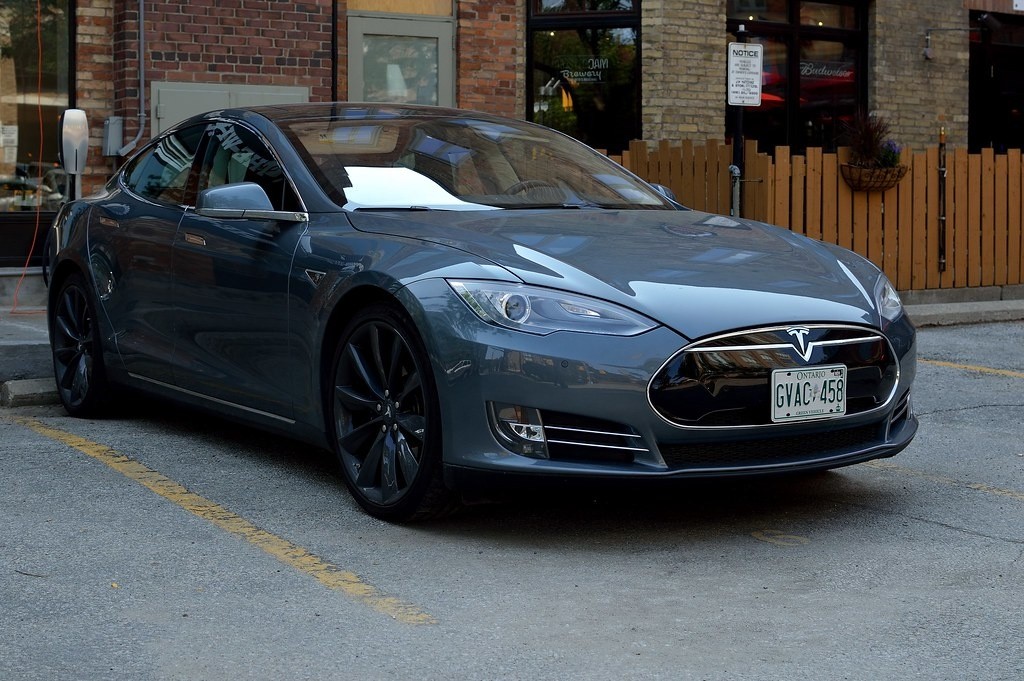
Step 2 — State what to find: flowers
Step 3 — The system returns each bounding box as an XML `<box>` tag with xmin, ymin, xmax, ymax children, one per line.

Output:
<box><xmin>839</xmin><ymin>104</ymin><xmax>902</xmax><ymax>167</ymax></box>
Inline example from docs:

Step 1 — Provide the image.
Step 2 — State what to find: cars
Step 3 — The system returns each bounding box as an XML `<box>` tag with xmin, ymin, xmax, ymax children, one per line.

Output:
<box><xmin>43</xmin><ymin>103</ymin><xmax>923</xmax><ymax>528</ymax></box>
<box><xmin>745</xmin><ymin>55</ymin><xmax>867</xmax><ymax>131</ymax></box>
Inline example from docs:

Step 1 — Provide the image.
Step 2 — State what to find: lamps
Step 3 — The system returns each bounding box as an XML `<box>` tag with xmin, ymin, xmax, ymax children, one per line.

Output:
<box><xmin>923</xmin><ymin>14</ymin><xmax>1003</xmax><ymax>59</ymax></box>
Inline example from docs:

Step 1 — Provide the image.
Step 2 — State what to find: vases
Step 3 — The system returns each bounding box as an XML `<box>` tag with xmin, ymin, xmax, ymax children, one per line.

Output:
<box><xmin>841</xmin><ymin>163</ymin><xmax>908</xmax><ymax>192</ymax></box>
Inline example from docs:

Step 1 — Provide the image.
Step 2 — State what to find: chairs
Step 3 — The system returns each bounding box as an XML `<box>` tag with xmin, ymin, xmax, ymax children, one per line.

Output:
<box><xmin>413</xmin><ymin>147</ymin><xmax>462</xmax><ymax>196</ymax></box>
